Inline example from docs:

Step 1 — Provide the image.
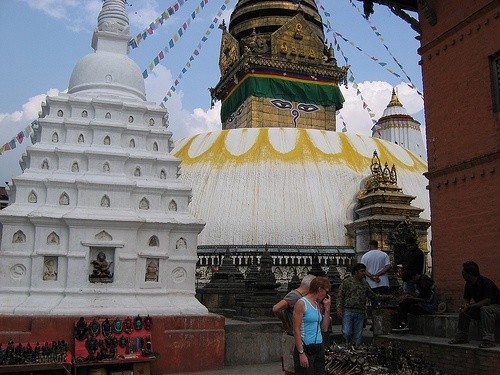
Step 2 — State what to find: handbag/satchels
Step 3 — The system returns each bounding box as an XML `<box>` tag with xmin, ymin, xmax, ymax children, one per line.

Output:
<box><xmin>306</xmin><ymin>345</ymin><xmax>317</xmax><ymax>355</ymax></box>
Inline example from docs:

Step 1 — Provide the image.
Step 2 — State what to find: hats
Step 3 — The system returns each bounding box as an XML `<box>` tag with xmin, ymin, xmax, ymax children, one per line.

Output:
<box><xmin>368</xmin><ymin>240</ymin><xmax>377</xmax><ymax>247</ymax></box>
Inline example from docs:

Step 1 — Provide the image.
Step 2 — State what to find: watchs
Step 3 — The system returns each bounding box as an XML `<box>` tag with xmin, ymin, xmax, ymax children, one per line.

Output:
<box><xmin>298</xmin><ymin>349</ymin><xmax>304</xmax><ymax>354</ymax></box>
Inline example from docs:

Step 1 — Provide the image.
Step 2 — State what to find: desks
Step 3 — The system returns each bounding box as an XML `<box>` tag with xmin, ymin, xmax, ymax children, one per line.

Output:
<box><xmin>0</xmin><ymin>341</ymin><xmax>73</xmax><ymax>375</ymax></box>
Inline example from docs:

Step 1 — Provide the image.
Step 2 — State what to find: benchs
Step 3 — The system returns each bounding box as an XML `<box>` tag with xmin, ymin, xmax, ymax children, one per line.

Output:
<box><xmin>408</xmin><ymin>313</ymin><xmax>500</xmax><ymax>343</ymax></box>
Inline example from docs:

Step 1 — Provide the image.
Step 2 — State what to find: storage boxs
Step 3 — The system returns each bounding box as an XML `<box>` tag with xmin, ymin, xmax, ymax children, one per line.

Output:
<box><xmin>132</xmin><ymin>362</ymin><xmax>150</xmax><ymax>375</ymax></box>
<box><xmin>109</xmin><ymin>371</ymin><xmax>123</xmax><ymax>375</ymax></box>
<box><xmin>122</xmin><ymin>370</ymin><xmax>133</xmax><ymax>375</ymax></box>
<box><xmin>89</xmin><ymin>368</ymin><xmax>107</xmax><ymax>375</ymax></box>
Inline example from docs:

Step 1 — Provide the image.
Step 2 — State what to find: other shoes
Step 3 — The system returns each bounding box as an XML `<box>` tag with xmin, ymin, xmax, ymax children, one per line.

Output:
<box><xmin>388</xmin><ymin>331</ymin><xmax>406</xmax><ymax>335</ymax></box>
<box><xmin>392</xmin><ymin>323</ymin><xmax>409</xmax><ymax>331</ymax></box>
<box><xmin>449</xmin><ymin>337</ymin><xmax>469</xmax><ymax>344</ymax></box>
<box><xmin>479</xmin><ymin>340</ymin><xmax>494</xmax><ymax>348</ymax></box>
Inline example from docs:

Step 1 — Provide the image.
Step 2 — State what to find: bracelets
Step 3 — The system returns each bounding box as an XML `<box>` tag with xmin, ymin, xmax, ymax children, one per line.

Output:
<box><xmin>378</xmin><ymin>270</ymin><xmax>382</xmax><ymax>274</ymax></box>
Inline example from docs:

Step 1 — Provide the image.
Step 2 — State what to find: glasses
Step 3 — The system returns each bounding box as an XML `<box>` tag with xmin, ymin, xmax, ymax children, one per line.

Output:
<box><xmin>321</xmin><ymin>287</ymin><xmax>328</xmax><ymax>294</ymax></box>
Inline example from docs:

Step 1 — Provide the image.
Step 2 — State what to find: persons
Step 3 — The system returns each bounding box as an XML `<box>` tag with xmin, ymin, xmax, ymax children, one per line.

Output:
<box><xmin>392</xmin><ymin>268</ymin><xmax>438</xmax><ymax>333</ymax></box>
<box><xmin>447</xmin><ymin>261</ymin><xmax>500</xmax><ymax>348</ymax></box>
<box><xmin>401</xmin><ymin>238</ymin><xmax>425</xmax><ymax>294</ymax></box>
<box><xmin>292</xmin><ymin>277</ymin><xmax>331</xmax><ymax>375</ymax></box>
<box><xmin>212</xmin><ymin>265</ymin><xmax>218</xmax><ymax>272</ymax></box>
<box><xmin>362</xmin><ymin>240</ymin><xmax>392</xmax><ymax>331</ymax></box>
<box><xmin>92</xmin><ymin>251</ymin><xmax>110</xmax><ymax>276</ymax></box>
<box><xmin>44</xmin><ymin>261</ymin><xmax>56</xmax><ymax>277</ymax></box>
<box><xmin>273</xmin><ymin>274</ymin><xmax>316</xmax><ymax>375</ymax></box>
<box><xmin>336</xmin><ymin>263</ymin><xmax>394</xmax><ymax>349</ymax></box>
